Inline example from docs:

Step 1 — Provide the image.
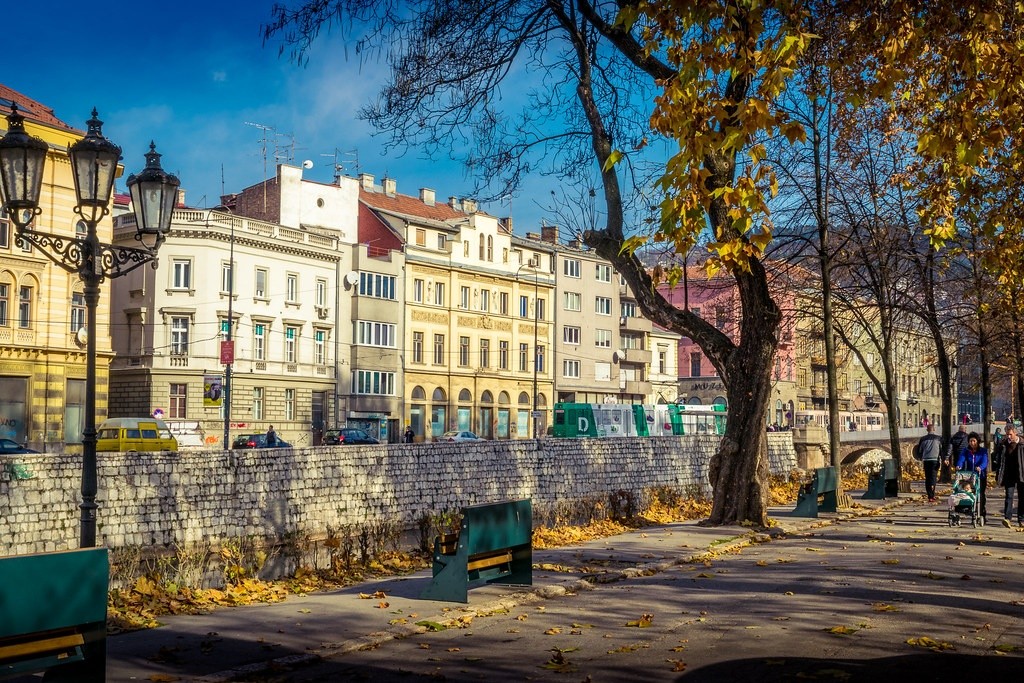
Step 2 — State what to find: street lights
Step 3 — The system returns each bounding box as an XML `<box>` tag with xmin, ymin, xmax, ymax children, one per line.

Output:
<box><xmin>515</xmin><ymin>264</ymin><xmax>538</xmax><ymax>439</ymax></box>
<box><xmin>0</xmin><ymin>99</ymin><xmax>181</xmax><ymax>549</ymax></box>
<box><xmin>205</xmin><ymin>204</ymin><xmax>234</xmax><ymax>450</ymax></box>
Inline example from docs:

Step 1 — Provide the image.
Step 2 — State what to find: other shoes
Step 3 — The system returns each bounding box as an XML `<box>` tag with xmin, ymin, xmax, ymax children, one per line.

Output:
<box><xmin>1002</xmin><ymin>519</ymin><xmax>1011</xmax><ymax>528</ymax></box>
<box><xmin>929</xmin><ymin>498</ymin><xmax>936</xmax><ymax>502</ymax></box>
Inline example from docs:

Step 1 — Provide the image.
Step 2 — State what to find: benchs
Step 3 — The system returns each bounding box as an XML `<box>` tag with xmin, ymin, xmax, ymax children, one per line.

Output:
<box><xmin>862</xmin><ymin>458</ymin><xmax>898</xmax><ymax>499</ymax></box>
<box><xmin>418</xmin><ymin>498</ymin><xmax>532</xmax><ymax>603</ymax></box>
<box><xmin>0</xmin><ymin>546</ymin><xmax>109</xmax><ymax>683</ymax></box>
<box><xmin>792</xmin><ymin>467</ymin><xmax>837</xmax><ymax>517</ymax></box>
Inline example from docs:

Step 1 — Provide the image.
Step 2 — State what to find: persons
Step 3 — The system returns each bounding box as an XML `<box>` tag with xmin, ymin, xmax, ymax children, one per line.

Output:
<box><xmin>945</xmin><ymin>425</ymin><xmax>969</xmax><ymax>471</ymax></box>
<box><xmin>766</xmin><ymin>421</ymin><xmax>791</xmax><ymax>432</ymax></box>
<box><xmin>265</xmin><ymin>425</ymin><xmax>276</xmax><ymax>448</ymax></box>
<box><xmin>918</xmin><ymin>425</ymin><xmax>947</xmax><ymax>502</ymax></box>
<box><xmin>993</xmin><ymin>427</ymin><xmax>1003</xmax><ymax>445</ymax></box>
<box><xmin>966</xmin><ymin>414</ymin><xmax>973</xmax><ymax>425</ymax></box>
<box><xmin>995</xmin><ymin>425</ymin><xmax>1024</xmax><ymax>529</ymax></box>
<box><xmin>962</xmin><ymin>482</ymin><xmax>973</xmax><ymax>493</ymax></box>
<box><xmin>991</xmin><ymin>410</ymin><xmax>996</xmax><ymax>424</ymax></box>
<box><xmin>405</xmin><ymin>426</ymin><xmax>415</xmax><ymax>443</ymax></box>
<box><xmin>1007</xmin><ymin>416</ymin><xmax>1013</xmax><ymax>424</ymax></box>
<box><xmin>956</xmin><ymin>431</ymin><xmax>988</xmax><ymax>525</ymax></box>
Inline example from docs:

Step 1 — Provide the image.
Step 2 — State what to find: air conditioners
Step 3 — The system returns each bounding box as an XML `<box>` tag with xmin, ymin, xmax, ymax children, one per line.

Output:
<box><xmin>528</xmin><ymin>258</ymin><xmax>538</xmax><ymax>267</ymax></box>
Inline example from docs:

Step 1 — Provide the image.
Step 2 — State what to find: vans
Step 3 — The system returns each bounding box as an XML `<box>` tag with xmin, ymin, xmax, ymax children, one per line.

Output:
<box><xmin>96</xmin><ymin>417</ymin><xmax>179</xmax><ymax>452</ymax></box>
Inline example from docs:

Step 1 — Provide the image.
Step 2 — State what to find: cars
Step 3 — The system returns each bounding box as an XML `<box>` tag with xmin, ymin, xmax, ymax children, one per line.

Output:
<box><xmin>435</xmin><ymin>431</ymin><xmax>487</xmax><ymax>442</ymax></box>
<box><xmin>232</xmin><ymin>433</ymin><xmax>293</xmax><ymax>449</ymax></box>
<box><xmin>0</xmin><ymin>438</ymin><xmax>41</xmax><ymax>454</ymax></box>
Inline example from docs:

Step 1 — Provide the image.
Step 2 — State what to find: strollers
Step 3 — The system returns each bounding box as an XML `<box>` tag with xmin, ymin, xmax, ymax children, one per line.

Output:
<box><xmin>948</xmin><ymin>466</ymin><xmax>985</xmax><ymax>529</ymax></box>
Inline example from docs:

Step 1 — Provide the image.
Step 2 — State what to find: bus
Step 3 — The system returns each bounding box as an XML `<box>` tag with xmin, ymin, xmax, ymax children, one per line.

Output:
<box><xmin>794</xmin><ymin>410</ymin><xmax>884</xmax><ymax>433</ymax></box>
<box><xmin>552</xmin><ymin>402</ymin><xmax>728</xmax><ymax>439</ymax></box>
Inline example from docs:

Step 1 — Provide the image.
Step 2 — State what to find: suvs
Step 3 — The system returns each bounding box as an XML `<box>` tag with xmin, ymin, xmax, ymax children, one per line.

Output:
<box><xmin>321</xmin><ymin>427</ymin><xmax>381</xmax><ymax>446</ymax></box>
